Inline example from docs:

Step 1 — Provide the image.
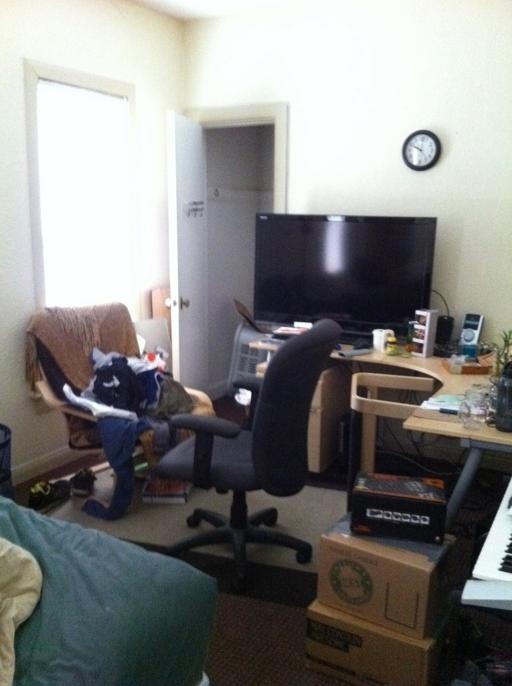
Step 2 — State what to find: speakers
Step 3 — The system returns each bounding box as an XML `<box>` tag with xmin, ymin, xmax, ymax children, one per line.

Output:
<box><xmin>436</xmin><ymin>315</ymin><xmax>454</xmax><ymax>344</ymax></box>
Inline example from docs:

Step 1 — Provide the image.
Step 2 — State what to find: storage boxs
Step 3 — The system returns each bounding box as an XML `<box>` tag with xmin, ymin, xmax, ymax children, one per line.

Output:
<box><xmin>306</xmin><ymin>598</ymin><xmax>461</xmax><ymax>686</ymax></box>
<box><xmin>318</xmin><ymin>514</ymin><xmax>457</xmax><ymax>639</ymax></box>
<box><xmin>350</xmin><ymin>473</ymin><xmax>446</xmax><ymax>545</ymax></box>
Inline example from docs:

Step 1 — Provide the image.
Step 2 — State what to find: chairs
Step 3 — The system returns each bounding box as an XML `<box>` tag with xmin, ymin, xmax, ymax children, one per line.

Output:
<box><xmin>149</xmin><ymin>319</ymin><xmax>344</xmax><ymax>591</ymax></box>
<box><xmin>25</xmin><ymin>303</ymin><xmax>216</xmax><ymax>495</ymax></box>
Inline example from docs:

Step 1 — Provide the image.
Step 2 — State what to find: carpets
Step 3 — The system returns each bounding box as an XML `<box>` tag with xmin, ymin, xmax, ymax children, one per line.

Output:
<box><xmin>204</xmin><ymin>593</ymin><xmax>307</xmax><ymax>686</ymax></box>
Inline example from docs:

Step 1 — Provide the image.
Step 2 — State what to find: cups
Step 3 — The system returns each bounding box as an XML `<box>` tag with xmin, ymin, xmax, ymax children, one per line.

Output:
<box><xmin>371</xmin><ymin>327</ymin><xmax>395</xmax><ymax>354</ymax></box>
<box><xmin>457</xmin><ymin>386</ymin><xmax>488</xmax><ymax>432</ymax></box>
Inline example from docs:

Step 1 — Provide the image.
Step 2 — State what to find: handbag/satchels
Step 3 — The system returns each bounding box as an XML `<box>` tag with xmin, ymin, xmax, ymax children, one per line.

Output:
<box><xmin>145</xmin><ymin>370</ymin><xmax>193</xmax><ymax>419</ymax></box>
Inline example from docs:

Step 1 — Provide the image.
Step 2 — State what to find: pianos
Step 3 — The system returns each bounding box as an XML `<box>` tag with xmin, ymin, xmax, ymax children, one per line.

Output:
<box><xmin>458</xmin><ymin>470</ymin><xmax>512</xmax><ymax>620</ymax></box>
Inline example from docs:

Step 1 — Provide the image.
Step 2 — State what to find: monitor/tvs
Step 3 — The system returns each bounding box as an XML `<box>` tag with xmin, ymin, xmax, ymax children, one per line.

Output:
<box><xmin>253</xmin><ymin>212</ymin><xmax>437</xmax><ymax>333</ymax></box>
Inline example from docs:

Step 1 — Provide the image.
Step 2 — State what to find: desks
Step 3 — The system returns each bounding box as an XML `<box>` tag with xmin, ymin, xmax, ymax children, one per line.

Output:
<box><xmin>248</xmin><ymin>336</ymin><xmax>511</xmax><ymax>530</ymax></box>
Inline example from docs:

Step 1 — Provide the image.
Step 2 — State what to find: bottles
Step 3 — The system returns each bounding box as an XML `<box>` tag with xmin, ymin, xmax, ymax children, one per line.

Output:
<box><xmin>384</xmin><ymin>336</ymin><xmax>398</xmax><ymax>358</ymax></box>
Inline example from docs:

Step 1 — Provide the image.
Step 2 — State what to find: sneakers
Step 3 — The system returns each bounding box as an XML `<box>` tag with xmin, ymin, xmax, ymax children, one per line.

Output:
<box><xmin>70</xmin><ymin>467</ymin><xmax>96</xmax><ymax>497</ymax></box>
<box><xmin>29</xmin><ymin>479</ymin><xmax>69</xmax><ymax>513</ymax></box>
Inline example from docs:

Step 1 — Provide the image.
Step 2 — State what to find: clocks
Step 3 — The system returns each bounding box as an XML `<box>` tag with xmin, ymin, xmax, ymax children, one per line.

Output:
<box><xmin>402</xmin><ymin>130</ymin><xmax>441</xmax><ymax>171</ymax></box>
